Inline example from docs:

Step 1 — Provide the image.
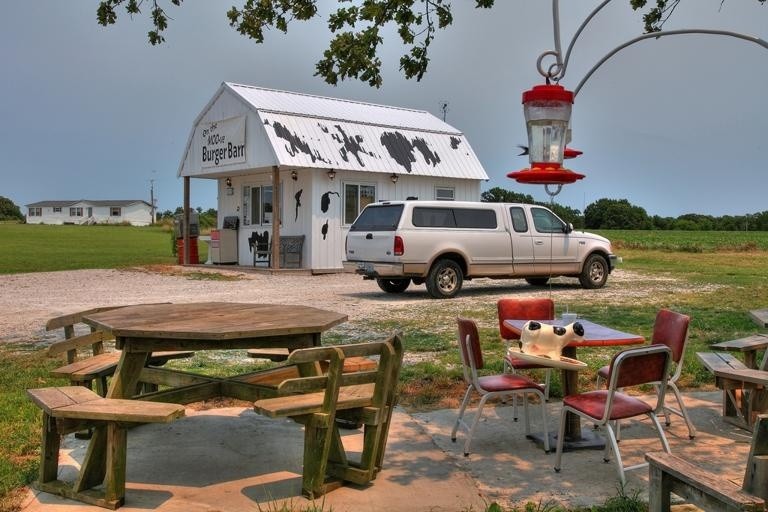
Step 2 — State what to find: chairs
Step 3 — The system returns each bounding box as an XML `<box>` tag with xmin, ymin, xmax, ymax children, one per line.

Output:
<box><xmin>451</xmin><ymin>317</ymin><xmax>550</xmax><ymax>456</ymax></box>
<box><xmin>499</xmin><ymin>298</ymin><xmax>554</xmax><ymax>421</ymax></box>
<box><xmin>598</xmin><ymin>308</ymin><xmax>697</xmax><ymax>440</ymax></box>
<box><xmin>554</xmin><ymin>344</ymin><xmax>671</xmax><ymax>494</ymax></box>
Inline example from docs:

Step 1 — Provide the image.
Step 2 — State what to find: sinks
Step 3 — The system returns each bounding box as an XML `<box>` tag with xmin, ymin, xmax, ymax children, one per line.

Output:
<box><xmin>198</xmin><ymin>235</ymin><xmax>211</xmax><ymax>243</ymax></box>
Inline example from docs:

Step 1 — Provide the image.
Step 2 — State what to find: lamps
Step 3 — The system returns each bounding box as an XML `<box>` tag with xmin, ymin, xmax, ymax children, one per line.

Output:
<box><xmin>292</xmin><ymin>170</ymin><xmax>298</xmax><ymax>180</ymax></box>
<box><xmin>328</xmin><ymin>169</ymin><xmax>336</xmax><ymax>180</ymax></box>
<box><xmin>391</xmin><ymin>173</ymin><xmax>399</xmax><ymax>184</ymax></box>
<box><xmin>226</xmin><ymin>177</ymin><xmax>231</xmax><ymax>186</ymax></box>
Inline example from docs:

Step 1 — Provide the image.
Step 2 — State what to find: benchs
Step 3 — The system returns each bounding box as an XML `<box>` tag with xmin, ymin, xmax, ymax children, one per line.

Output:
<box><xmin>247</xmin><ymin>349</ymin><xmax>381</xmax><ymax>434</ymax></box>
<box><xmin>26</xmin><ymin>383</ymin><xmax>185</xmax><ymax>508</ymax></box>
<box><xmin>43</xmin><ymin>301</ymin><xmax>192</xmax><ymax>445</ymax></box>
<box><xmin>645</xmin><ymin>333</ymin><xmax>768</xmax><ymax>511</ymax></box>
<box><xmin>255</xmin><ymin>336</ymin><xmax>404</xmax><ymax>500</ymax></box>
<box><xmin>252</xmin><ymin>234</ymin><xmax>305</xmax><ymax>268</ymax></box>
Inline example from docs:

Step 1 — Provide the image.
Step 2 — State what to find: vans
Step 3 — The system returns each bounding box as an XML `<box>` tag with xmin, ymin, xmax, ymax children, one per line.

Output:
<box><xmin>341</xmin><ymin>201</ymin><xmax>622</xmax><ymax>299</ymax></box>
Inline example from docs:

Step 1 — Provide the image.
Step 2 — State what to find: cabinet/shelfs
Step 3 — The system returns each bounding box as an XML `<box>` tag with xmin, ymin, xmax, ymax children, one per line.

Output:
<box><xmin>210</xmin><ymin>228</ymin><xmax>238</xmax><ymax>266</ymax></box>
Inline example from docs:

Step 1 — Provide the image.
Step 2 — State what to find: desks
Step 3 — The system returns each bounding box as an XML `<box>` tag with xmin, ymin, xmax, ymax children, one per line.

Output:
<box><xmin>503</xmin><ymin>320</ymin><xmax>645</xmax><ymax>452</ymax></box>
<box><xmin>748</xmin><ymin>307</ymin><xmax>768</xmax><ymax>371</ymax></box>
<box><xmin>73</xmin><ymin>302</ymin><xmax>348</xmax><ymax>498</ymax></box>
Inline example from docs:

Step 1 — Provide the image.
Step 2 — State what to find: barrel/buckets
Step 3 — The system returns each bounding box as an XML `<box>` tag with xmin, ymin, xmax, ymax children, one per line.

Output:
<box><xmin>173</xmin><ymin>212</ymin><xmax>200</xmax><ymax>239</ymax></box>
<box><xmin>176</xmin><ymin>238</ymin><xmax>199</xmax><ymax>266</ymax></box>
